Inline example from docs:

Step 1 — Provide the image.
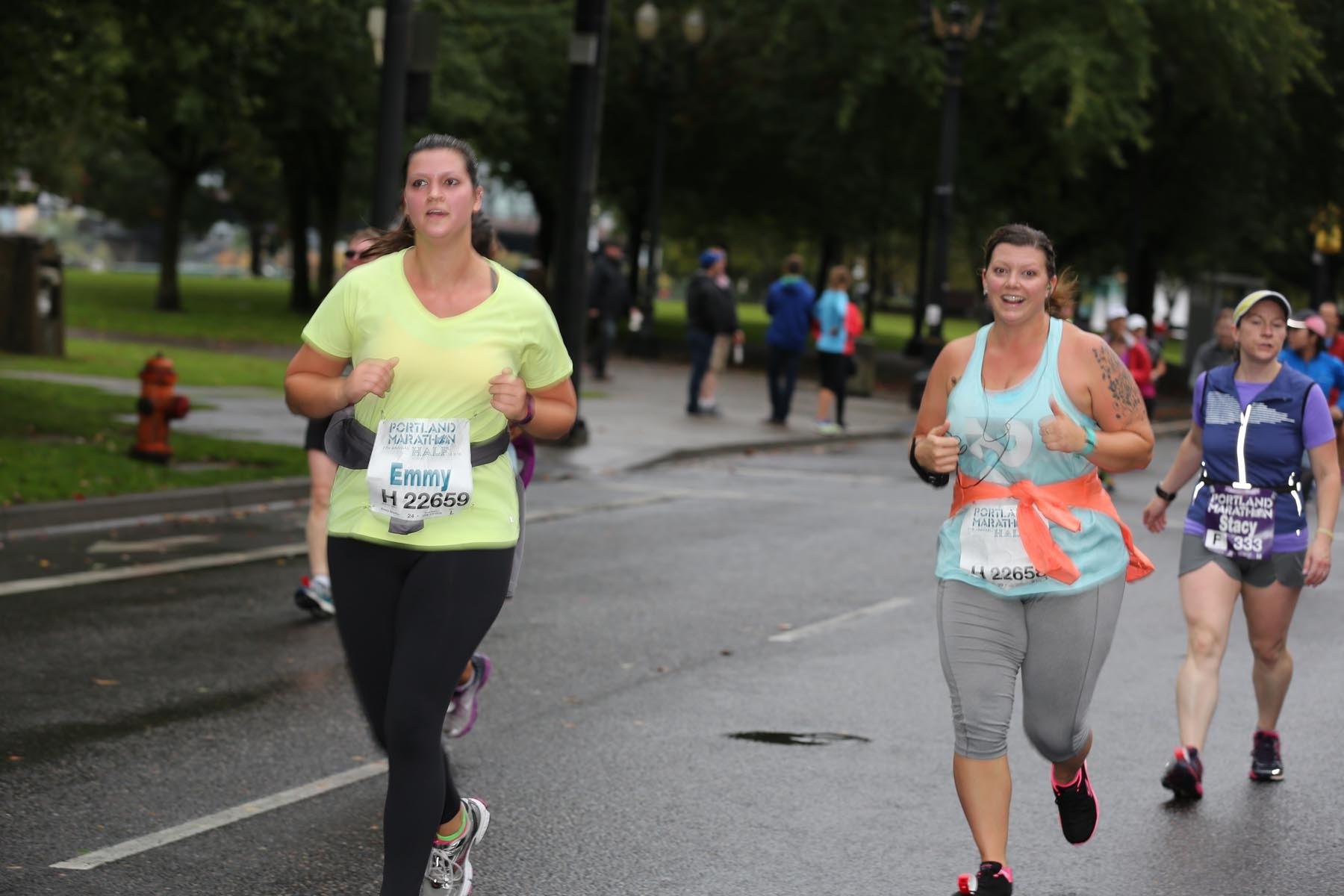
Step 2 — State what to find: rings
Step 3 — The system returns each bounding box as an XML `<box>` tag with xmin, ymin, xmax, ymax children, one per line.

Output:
<box><xmin>1045</xmin><ymin>427</ymin><xmax>1050</xmax><ymax>435</ymax></box>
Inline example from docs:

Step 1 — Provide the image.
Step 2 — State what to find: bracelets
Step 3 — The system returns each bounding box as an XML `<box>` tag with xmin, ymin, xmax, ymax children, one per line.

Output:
<box><xmin>1074</xmin><ymin>426</ymin><xmax>1096</xmax><ymax>459</ymax></box>
<box><xmin>1315</xmin><ymin>527</ymin><xmax>1334</xmax><ymax>540</ymax></box>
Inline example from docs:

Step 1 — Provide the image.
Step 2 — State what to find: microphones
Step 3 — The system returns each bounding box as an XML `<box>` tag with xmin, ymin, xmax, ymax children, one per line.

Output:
<box><xmin>1048</xmin><ymin>285</ymin><xmax>1052</xmax><ymax>292</ymax></box>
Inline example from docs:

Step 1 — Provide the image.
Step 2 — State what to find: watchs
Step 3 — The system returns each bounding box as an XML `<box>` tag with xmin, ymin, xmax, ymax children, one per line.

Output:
<box><xmin>1155</xmin><ymin>482</ymin><xmax>1175</xmax><ymax>503</ymax></box>
<box><xmin>509</xmin><ymin>392</ymin><xmax>536</xmax><ymax>425</ymax></box>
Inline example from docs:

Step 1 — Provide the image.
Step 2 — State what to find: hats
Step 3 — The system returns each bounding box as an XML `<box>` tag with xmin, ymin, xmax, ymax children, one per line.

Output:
<box><xmin>1106</xmin><ymin>305</ymin><xmax>1129</xmax><ymax>323</ymax></box>
<box><xmin>1232</xmin><ymin>289</ymin><xmax>1292</xmax><ymax>326</ymax></box>
<box><xmin>1286</xmin><ymin>308</ymin><xmax>1326</xmax><ymax>336</ymax></box>
<box><xmin>698</xmin><ymin>251</ymin><xmax>726</xmax><ymax>267</ymax></box>
<box><xmin>1124</xmin><ymin>314</ymin><xmax>1148</xmax><ymax>332</ymax></box>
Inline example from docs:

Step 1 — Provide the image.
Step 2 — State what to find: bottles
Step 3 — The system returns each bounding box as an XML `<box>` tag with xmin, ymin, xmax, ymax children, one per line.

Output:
<box><xmin>733</xmin><ymin>344</ymin><xmax>744</xmax><ymax>363</ymax></box>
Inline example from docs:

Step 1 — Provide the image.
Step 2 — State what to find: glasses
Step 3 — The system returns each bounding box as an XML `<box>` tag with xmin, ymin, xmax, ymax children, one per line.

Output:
<box><xmin>344</xmin><ymin>250</ymin><xmax>369</xmax><ymax>260</ymax></box>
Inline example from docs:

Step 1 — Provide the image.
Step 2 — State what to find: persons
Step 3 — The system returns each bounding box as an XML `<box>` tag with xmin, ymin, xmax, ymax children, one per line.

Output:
<box><xmin>1140</xmin><ymin>290</ymin><xmax>1343</xmax><ymax>802</ymax></box>
<box><xmin>285</xmin><ymin>133</ymin><xmax>581</xmax><ymax>895</ymax></box>
<box><xmin>294</xmin><ymin>217</ymin><xmax>1344</xmax><ymax>736</ymax></box>
<box><xmin>904</xmin><ymin>223</ymin><xmax>1154</xmax><ymax>896</ymax></box>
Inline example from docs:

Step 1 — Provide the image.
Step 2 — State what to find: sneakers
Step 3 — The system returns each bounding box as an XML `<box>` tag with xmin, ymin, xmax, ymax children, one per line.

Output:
<box><xmin>1249</xmin><ymin>729</ymin><xmax>1285</xmax><ymax>782</ymax></box>
<box><xmin>952</xmin><ymin>860</ymin><xmax>1012</xmax><ymax>896</ymax></box>
<box><xmin>293</xmin><ymin>576</ymin><xmax>336</xmax><ymax>620</ymax></box>
<box><xmin>417</xmin><ymin>797</ymin><xmax>491</xmax><ymax>896</ymax></box>
<box><xmin>1050</xmin><ymin>759</ymin><xmax>1101</xmax><ymax>846</ymax></box>
<box><xmin>1161</xmin><ymin>745</ymin><xmax>1204</xmax><ymax>801</ymax></box>
<box><xmin>440</xmin><ymin>652</ymin><xmax>492</xmax><ymax>739</ymax></box>
